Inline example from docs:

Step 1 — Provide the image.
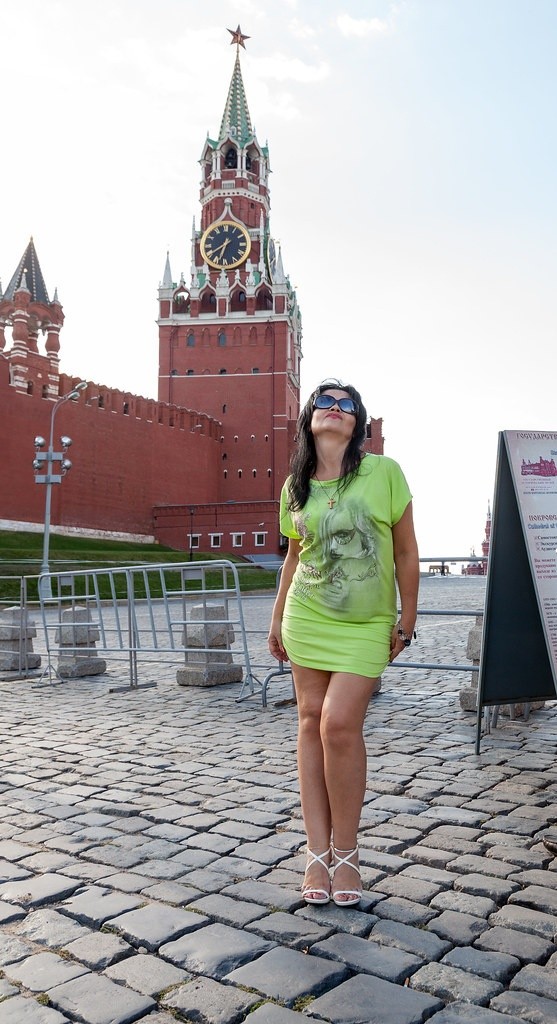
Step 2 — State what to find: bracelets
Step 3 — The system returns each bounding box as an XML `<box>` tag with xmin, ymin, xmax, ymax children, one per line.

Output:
<box><xmin>397</xmin><ymin>620</ymin><xmax>416</xmax><ymax>647</ymax></box>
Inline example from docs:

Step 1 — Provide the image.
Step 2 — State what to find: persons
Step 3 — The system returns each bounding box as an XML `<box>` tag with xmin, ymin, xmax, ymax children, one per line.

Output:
<box><xmin>267</xmin><ymin>376</ymin><xmax>419</xmax><ymax>908</ymax></box>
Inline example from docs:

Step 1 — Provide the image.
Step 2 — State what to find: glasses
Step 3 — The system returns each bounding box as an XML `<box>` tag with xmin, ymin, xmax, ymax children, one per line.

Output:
<box><xmin>312</xmin><ymin>394</ymin><xmax>360</xmax><ymax>418</ymax></box>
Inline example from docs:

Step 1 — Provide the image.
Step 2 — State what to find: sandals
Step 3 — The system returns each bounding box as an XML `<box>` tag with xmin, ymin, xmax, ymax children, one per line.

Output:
<box><xmin>300</xmin><ymin>841</ymin><xmax>364</xmax><ymax>906</ymax></box>
